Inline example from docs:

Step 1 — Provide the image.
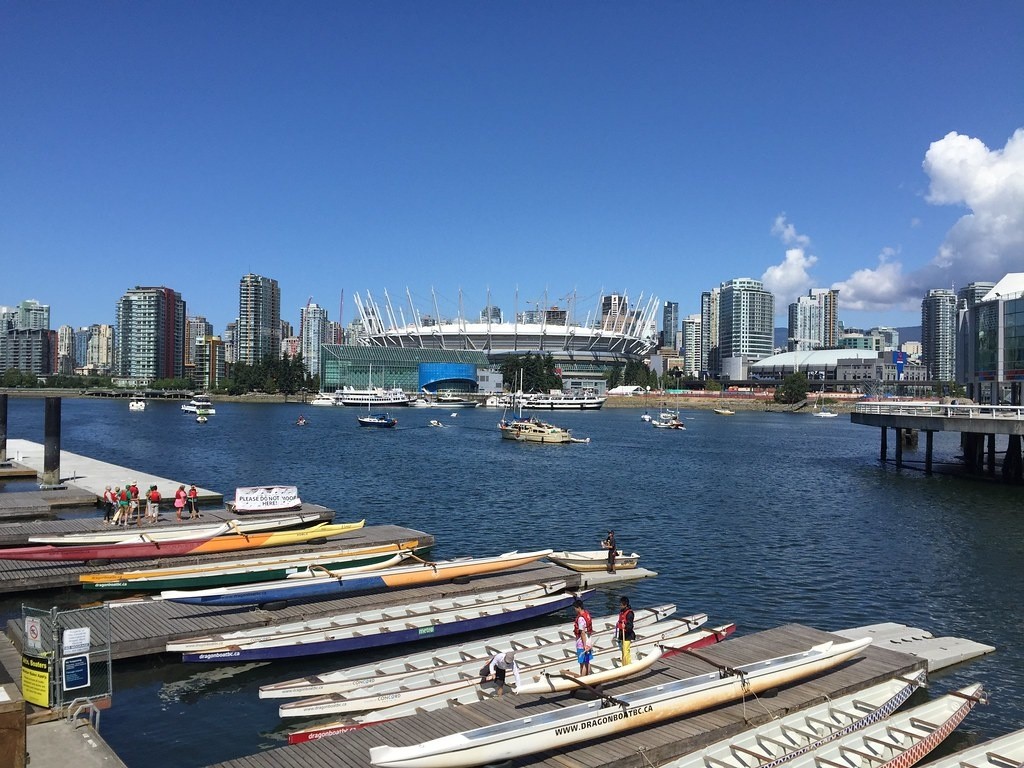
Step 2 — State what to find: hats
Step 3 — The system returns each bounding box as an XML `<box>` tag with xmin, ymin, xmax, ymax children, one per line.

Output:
<box><xmin>106</xmin><ymin>486</ymin><xmax>111</xmax><ymax>489</ymax></box>
<box><xmin>191</xmin><ymin>485</ymin><xmax>195</xmax><ymax>488</ymax></box>
<box><xmin>132</xmin><ymin>480</ymin><xmax>137</xmax><ymax>485</ymax></box>
<box><xmin>506</xmin><ymin>652</ymin><xmax>514</xmax><ymax>663</ymax></box>
<box><xmin>150</xmin><ymin>484</ymin><xmax>154</xmax><ymax>488</ymax></box>
<box><xmin>126</xmin><ymin>484</ymin><xmax>130</xmax><ymax>489</ymax></box>
<box><xmin>115</xmin><ymin>485</ymin><xmax>120</xmax><ymax>490</ymax></box>
<box><xmin>607</xmin><ymin>529</ymin><xmax>615</xmax><ymax>533</ymax></box>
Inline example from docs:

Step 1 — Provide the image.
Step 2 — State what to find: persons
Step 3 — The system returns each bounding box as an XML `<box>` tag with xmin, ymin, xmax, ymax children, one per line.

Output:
<box><xmin>574</xmin><ymin>599</ymin><xmax>593</xmax><ymax>676</ymax></box>
<box><xmin>119</xmin><ymin>484</ymin><xmax>131</xmax><ymax>526</ymax></box>
<box><xmin>601</xmin><ymin>530</ymin><xmax>616</xmax><ymax>574</ymax></box>
<box><xmin>103</xmin><ymin>486</ymin><xmax>113</xmax><ymax>523</ymax></box>
<box><xmin>173</xmin><ymin>485</ymin><xmax>187</xmax><ymax>522</ymax></box>
<box><xmin>146</xmin><ymin>484</ymin><xmax>162</xmax><ymax>523</ymax></box>
<box><xmin>480</xmin><ymin>652</ymin><xmax>521</xmax><ymax>696</ymax></box>
<box><xmin>112</xmin><ymin>487</ymin><xmax>120</xmax><ymax>515</ymax></box>
<box><xmin>187</xmin><ymin>483</ymin><xmax>201</xmax><ymax>519</ymax></box>
<box><xmin>129</xmin><ymin>480</ymin><xmax>139</xmax><ymax>521</ymax></box>
<box><xmin>299</xmin><ymin>414</ymin><xmax>303</xmax><ymax>422</ymax></box>
<box><xmin>615</xmin><ymin>596</ymin><xmax>635</xmax><ymax>667</ymax></box>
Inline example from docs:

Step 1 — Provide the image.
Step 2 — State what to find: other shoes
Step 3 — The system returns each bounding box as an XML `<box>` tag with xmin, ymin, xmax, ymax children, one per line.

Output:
<box><xmin>153</xmin><ymin>521</ymin><xmax>157</xmax><ymax>525</ymax></box>
<box><xmin>609</xmin><ymin>570</ymin><xmax>616</xmax><ymax>574</ymax></box>
<box><xmin>118</xmin><ymin>523</ymin><xmax>121</xmax><ymax>526</ymax></box>
<box><xmin>148</xmin><ymin>521</ymin><xmax>151</xmax><ymax>524</ymax></box>
<box><xmin>103</xmin><ymin>520</ymin><xmax>108</xmax><ymax>523</ymax></box>
<box><xmin>124</xmin><ymin>523</ymin><xmax>127</xmax><ymax>526</ymax></box>
<box><xmin>190</xmin><ymin>515</ymin><xmax>192</xmax><ymax>518</ymax></box>
<box><xmin>199</xmin><ymin>516</ymin><xmax>201</xmax><ymax>519</ymax></box>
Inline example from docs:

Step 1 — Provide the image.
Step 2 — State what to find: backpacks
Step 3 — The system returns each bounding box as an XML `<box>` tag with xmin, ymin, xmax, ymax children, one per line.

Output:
<box><xmin>121</xmin><ymin>490</ymin><xmax>127</xmax><ymax>501</ymax></box>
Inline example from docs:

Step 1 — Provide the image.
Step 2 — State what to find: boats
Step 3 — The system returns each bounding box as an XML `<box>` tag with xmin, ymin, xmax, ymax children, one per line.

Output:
<box><xmin>258</xmin><ymin>603</ymin><xmax>736</xmax><ymax>746</ymax></box>
<box><xmin>160</xmin><ymin>547</ymin><xmax>555</xmax><ymax>609</ymax></box>
<box><xmin>0</xmin><ymin>518</ymin><xmax>369</xmax><ymax>560</ymax></box>
<box><xmin>297</xmin><ymin>420</ymin><xmax>306</xmax><ymax>426</ymax></box>
<box><xmin>341</xmin><ymin>380</ymin><xmax>417</xmax><ymax>406</ymax></box>
<box><xmin>78</xmin><ymin>540</ymin><xmax>421</xmax><ymax>592</ymax></box>
<box><xmin>521</xmin><ymin>391</ymin><xmax>608</xmax><ymax>410</ymax></box>
<box><xmin>313</xmin><ymin>392</ymin><xmax>336</xmax><ymax>407</ymax></box>
<box><xmin>128</xmin><ymin>400</ymin><xmax>145</xmax><ymax>411</ymax></box>
<box><xmin>546</xmin><ymin>549</ymin><xmax>640</xmax><ymax>573</ymax></box>
<box><xmin>196</xmin><ymin>415</ymin><xmax>208</xmax><ymax>424</ymax></box>
<box><xmin>181</xmin><ymin>394</ymin><xmax>217</xmax><ymax>415</ymax></box>
<box><xmin>370</xmin><ymin>635</ymin><xmax>874</xmax><ymax>768</ymax></box>
<box><xmin>658</xmin><ymin>667</ymin><xmax>929</xmax><ymax>768</ymax></box>
<box><xmin>27</xmin><ymin>512</ymin><xmax>321</xmax><ymax>547</ymax></box>
<box><xmin>406</xmin><ymin>399</ymin><xmax>487</xmax><ymax>407</ymax></box>
<box><xmin>917</xmin><ymin>727</ymin><xmax>1024</xmax><ymax>768</ymax></box>
<box><xmin>770</xmin><ymin>680</ymin><xmax>990</xmax><ymax>768</ymax></box>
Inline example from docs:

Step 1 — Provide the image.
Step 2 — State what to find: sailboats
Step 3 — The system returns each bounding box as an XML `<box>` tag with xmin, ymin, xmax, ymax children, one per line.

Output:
<box><xmin>714</xmin><ymin>388</ymin><xmax>735</xmax><ymax>415</ymax></box>
<box><xmin>356</xmin><ymin>363</ymin><xmax>398</xmax><ymax>428</ymax></box>
<box><xmin>640</xmin><ymin>378</ymin><xmax>685</xmax><ymax>431</ymax></box>
<box><xmin>497</xmin><ymin>368</ymin><xmax>573</xmax><ymax>443</ymax></box>
<box><xmin>811</xmin><ymin>382</ymin><xmax>838</xmax><ymax>418</ymax></box>
